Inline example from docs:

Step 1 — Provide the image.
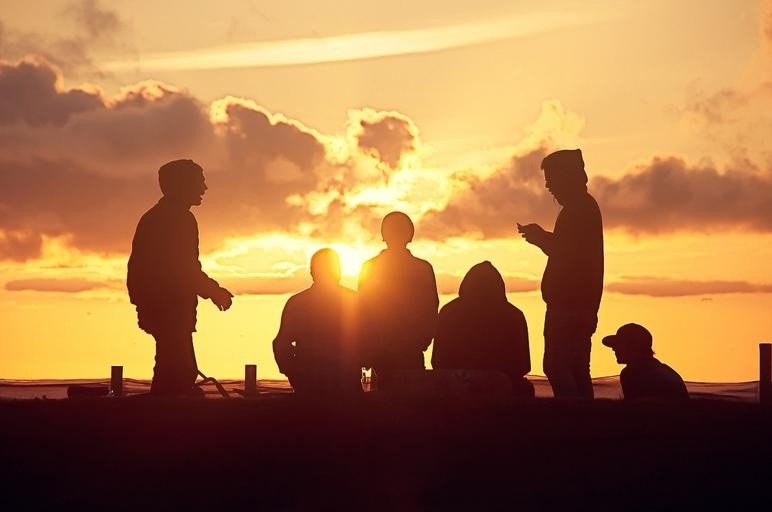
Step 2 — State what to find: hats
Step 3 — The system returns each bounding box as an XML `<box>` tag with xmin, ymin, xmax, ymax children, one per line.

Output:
<box><xmin>602</xmin><ymin>322</ymin><xmax>652</xmax><ymax>347</ymax></box>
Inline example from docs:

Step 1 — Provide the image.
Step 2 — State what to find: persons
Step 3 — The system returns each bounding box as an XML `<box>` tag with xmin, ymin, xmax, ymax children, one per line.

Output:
<box><xmin>272</xmin><ymin>248</ymin><xmax>362</xmax><ymax>394</ymax></box>
<box><xmin>601</xmin><ymin>324</ymin><xmax>690</xmax><ymax>403</ymax></box>
<box><xmin>126</xmin><ymin>160</ymin><xmax>234</xmax><ymax>393</ymax></box>
<box><xmin>518</xmin><ymin>149</ymin><xmax>605</xmax><ymax>399</ymax></box>
<box><xmin>357</xmin><ymin>211</ymin><xmax>438</xmax><ymax>392</ymax></box>
<box><xmin>431</xmin><ymin>261</ymin><xmax>535</xmax><ymax>399</ymax></box>
